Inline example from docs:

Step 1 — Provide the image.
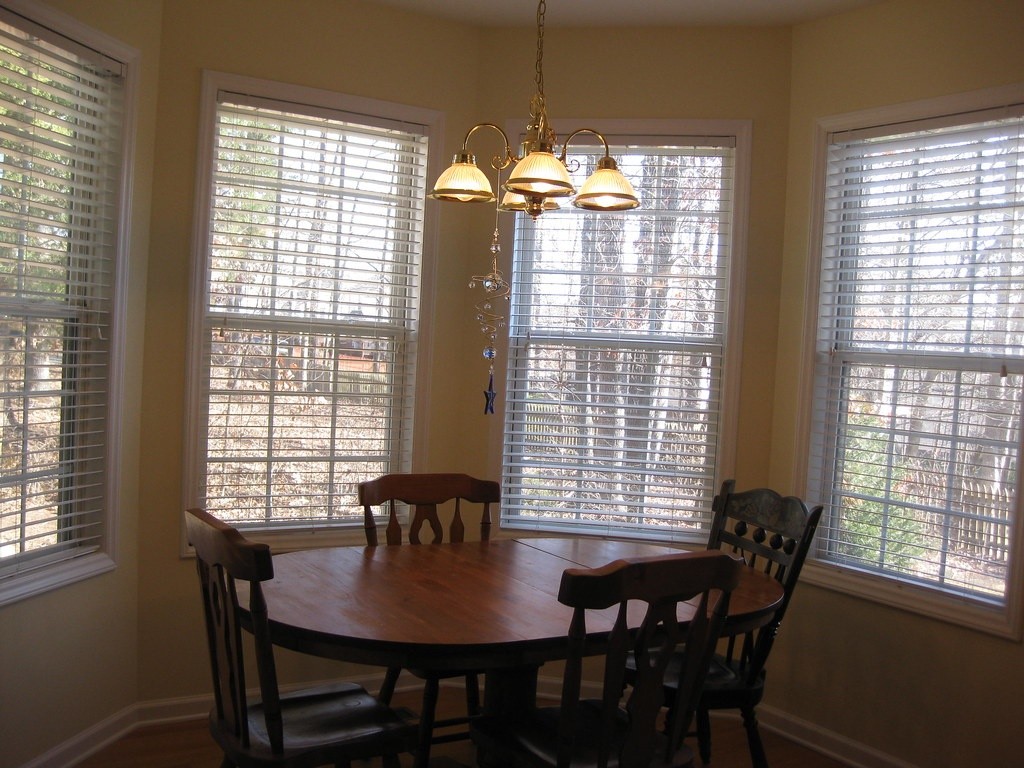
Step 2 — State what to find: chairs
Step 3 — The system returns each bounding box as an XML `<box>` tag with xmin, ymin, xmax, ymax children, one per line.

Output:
<box><xmin>184</xmin><ymin>471</ymin><xmax>823</xmax><ymax>767</ymax></box>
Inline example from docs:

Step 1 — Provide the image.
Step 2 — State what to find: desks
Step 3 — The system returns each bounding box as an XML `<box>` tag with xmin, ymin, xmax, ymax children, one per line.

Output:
<box><xmin>216</xmin><ymin>537</ymin><xmax>785</xmax><ymax>768</ymax></box>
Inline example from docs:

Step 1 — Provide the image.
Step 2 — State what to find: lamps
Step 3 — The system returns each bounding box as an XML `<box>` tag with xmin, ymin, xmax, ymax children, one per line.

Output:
<box><xmin>424</xmin><ymin>0</ymin><xmax>642</xmax><ymax>415</ymax></box>
